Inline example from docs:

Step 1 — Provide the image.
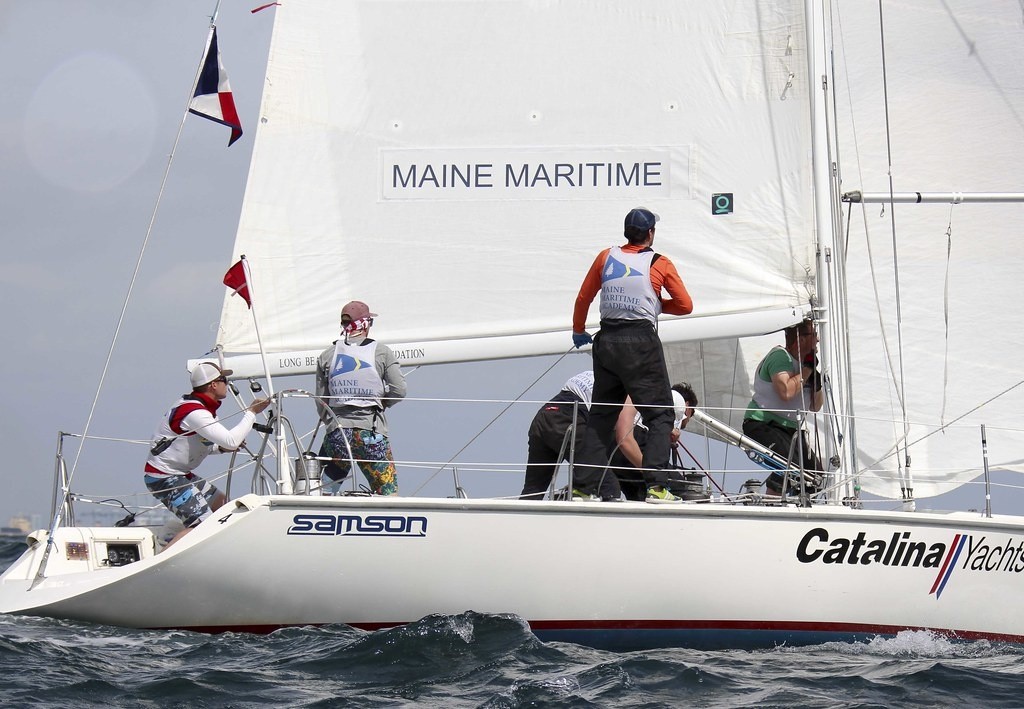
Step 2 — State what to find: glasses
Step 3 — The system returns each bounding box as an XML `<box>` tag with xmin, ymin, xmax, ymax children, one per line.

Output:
<box><xmin>794</xmin><ymin>332</ymin><xmax>818</xmax><ymax>338</ymax></box>
<box><xmin>214</xmin><ymin>377</ymin><xmax>226</xmax><ymax>385</ymax></box>
<box><xmin>691</xmin><ymin>409</ymin><xmax>694</xmax><ymax>417</ymax></box>
<box><xmin>366</xmin><ymin>318</ymin><xmax>373</xmax><ymax>327</ymax></box>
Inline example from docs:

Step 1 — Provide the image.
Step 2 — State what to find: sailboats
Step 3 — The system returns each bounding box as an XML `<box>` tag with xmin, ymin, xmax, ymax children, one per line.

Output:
<box><xmin>2</xmin><ymin>0</ymin><xmax>1024</xmax><ymax>650</ymax></box>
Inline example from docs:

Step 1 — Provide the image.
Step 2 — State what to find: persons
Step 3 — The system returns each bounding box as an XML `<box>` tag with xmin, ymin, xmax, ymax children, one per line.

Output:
<box><xmin>517</xmin><ymin>370</ymin><xmax>698</xmax><ymax>500</ymax></box>
<box><xmin>572</xmin><ymin>209</ymin><xmax>692</xmax><ymax>505</ymax></box>
<box><xmin>144</xmin><ymin>363</ymin><xmax>270</xmax><ymax>554</ymax></box>
<box><xmin>314</xmin><ymin>301</ymin><xmax>407</xmax><ymax>496</ymax></box>
<box><xmin>741</xmin><ymin>318</ymin><xmax>825</xmax><ymax>505</ymax></box>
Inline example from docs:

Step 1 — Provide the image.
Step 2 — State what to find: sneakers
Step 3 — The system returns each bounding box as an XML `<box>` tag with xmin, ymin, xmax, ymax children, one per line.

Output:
<box><xmin>645</xmin><ymin>487</ymin><xmax>683</xmax><ymax>505</ymax></box>
<box><xmin>572</xmin><ymin>488</ymin><xmax>602</xmax><ymax>501</ymax></box>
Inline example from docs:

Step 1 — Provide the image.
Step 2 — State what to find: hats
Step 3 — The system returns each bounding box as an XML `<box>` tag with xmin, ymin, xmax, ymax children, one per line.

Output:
<box><xmin>625</xmin><ymin>209</ymin><xmax>660</xmax><ymax>232</ymax></box>
<box><xmin>341</xmin><ymin>301</ymin><xmax>378</xmax><ymax>324</ymax></box>
<box><xmin>190</xmin><ymin>362</ymin><xmax>233</xmax><ymax>387</ymax></box>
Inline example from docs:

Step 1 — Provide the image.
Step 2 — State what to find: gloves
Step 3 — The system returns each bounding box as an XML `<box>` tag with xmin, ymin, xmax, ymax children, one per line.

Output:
<box><xmin>808</xmin><ymin>370</ymin><xmax>822</xmax><ymax>391</ymax></box>
<box><xmin>803</xmin><ymin>352</ymin><xmax>818</xmax><ymax>369</ymax></box>
<box><xmin>573</xmin><ymin>331</ymin><xmax>594</xmax><ymax>349</ymax></box>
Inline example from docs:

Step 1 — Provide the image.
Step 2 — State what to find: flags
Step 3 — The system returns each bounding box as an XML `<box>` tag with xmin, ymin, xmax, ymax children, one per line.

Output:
<box><xmin>223</xmin><ymin>259</ymin><xmax>251</xmax><ymax>310</ymax></box>
<box><xmin>187</xmin><ymin>24</ymin><xmax>243</xmax><ymax>147</ymax></box>
